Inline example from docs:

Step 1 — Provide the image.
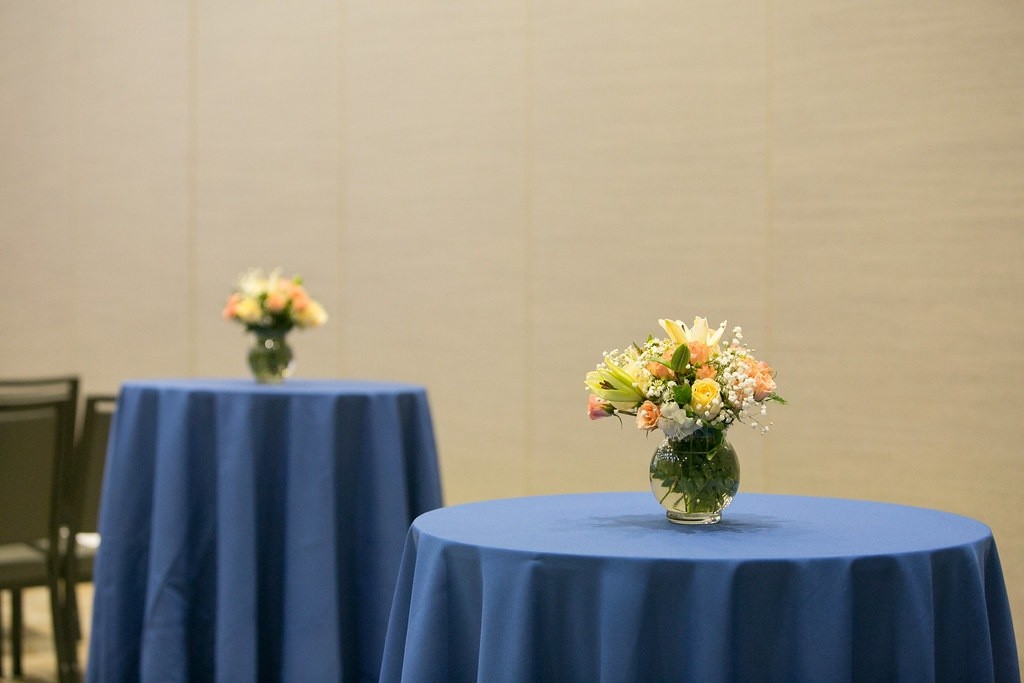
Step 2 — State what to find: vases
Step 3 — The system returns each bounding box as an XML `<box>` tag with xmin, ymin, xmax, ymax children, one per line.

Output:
<box><xmin>650</xmin><ymin>427</ymin><xmax>740</xmax><ymax>525</ymax></box>
<box><xmin>247</xmin><ymin>329</ymin><xmax>292</xmax><ymax>387</ymax></box>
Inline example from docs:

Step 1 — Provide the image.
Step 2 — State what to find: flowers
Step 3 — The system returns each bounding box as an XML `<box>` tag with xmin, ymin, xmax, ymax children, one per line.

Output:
<box><xmin>582</xmin><ymin>313</ymin><xmax>790</xmax><ymax>441</ymax></box>
<box><xmin>222</xmin><ymin>268</ymin><xmax>326</xmax><ymax>331</ymax></box>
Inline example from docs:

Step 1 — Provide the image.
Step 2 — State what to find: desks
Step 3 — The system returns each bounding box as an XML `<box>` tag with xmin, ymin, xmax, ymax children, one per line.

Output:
<box><xmin>88</xmin><ymin>381</ymin><xmax>444</xmax><ymax>683</ymax></box>
<box><xmin>378</xmin><ymin>491</ymin><xmax>1024</xmax><ymax>683</ymax></box>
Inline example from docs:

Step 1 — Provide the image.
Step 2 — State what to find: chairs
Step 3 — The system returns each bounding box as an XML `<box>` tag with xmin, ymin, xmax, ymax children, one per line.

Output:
<box><xmin>0</xmin><ymin>376</ymin><xmax>82</xmax><ymax>683</ymax></box>
<box><xmin>66</xmin><ymin>393</ymin><xmax>120</xmax><ymax>663</ymax></box>
